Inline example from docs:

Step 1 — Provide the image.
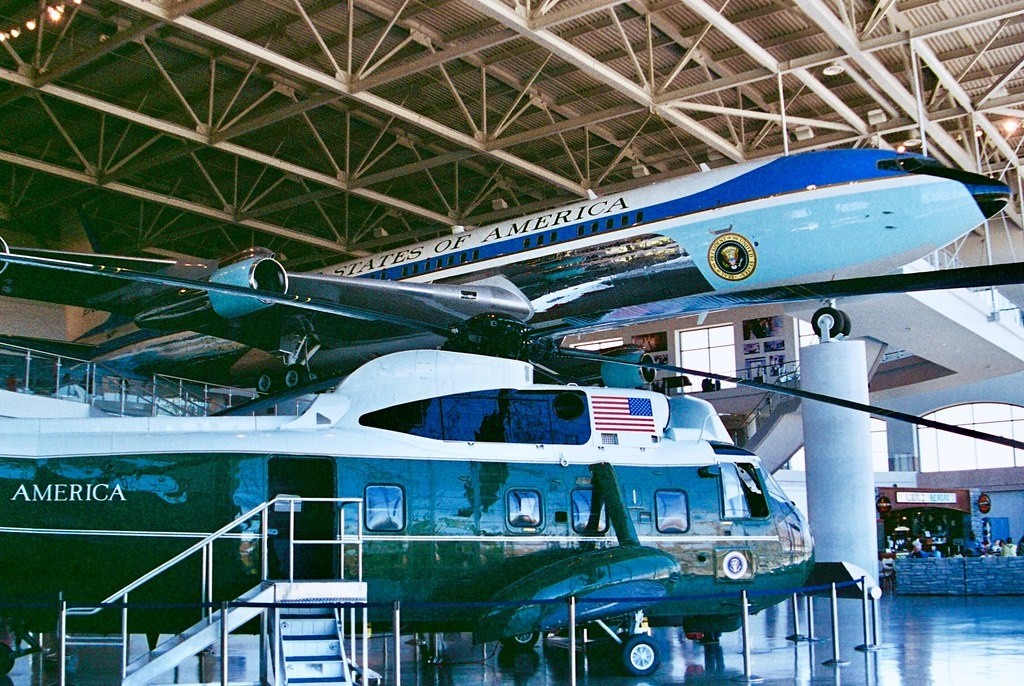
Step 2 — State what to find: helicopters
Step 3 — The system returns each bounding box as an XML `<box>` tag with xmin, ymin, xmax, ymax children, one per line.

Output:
<box><xmin>0</xmin><ymin>249</ymin><xmax>1023</xmax><ymax>680</ymax></box>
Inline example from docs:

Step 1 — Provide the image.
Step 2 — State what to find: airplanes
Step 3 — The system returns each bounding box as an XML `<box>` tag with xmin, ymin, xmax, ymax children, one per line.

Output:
<box><xmin>0</xmin><ymin>147</ymin><xmax>1018</xmax><ymax>394</ymax></box>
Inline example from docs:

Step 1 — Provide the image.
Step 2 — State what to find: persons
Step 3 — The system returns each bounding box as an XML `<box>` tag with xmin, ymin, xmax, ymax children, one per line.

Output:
<box><xmin>992</xmin><ymin>541</ymin><xmax>1005</xmax><ymax>552</ymax></box>
<box><xmin>1001</xmin><ymin>538</ymin><xmax>1018</xmax><ymax>557</ymax></box>
<box><xmin>907</xmin><ymin>542</ymin><xmax>928</xmax><ymax>558</ymax></box>
<box><xmin>1017</xmin><ymin>536</ymin><xmax>1024</xmax><ymax>556</ymax></box>
<box><xmin>981</xmin><ymin>530</ymin><xmax>991</xmax><ymax>552</ymax></box>
<box><xmin>965</xmin><ymin>530</ymin><xmax>982</xmax><ymax>557</ymax></box>
